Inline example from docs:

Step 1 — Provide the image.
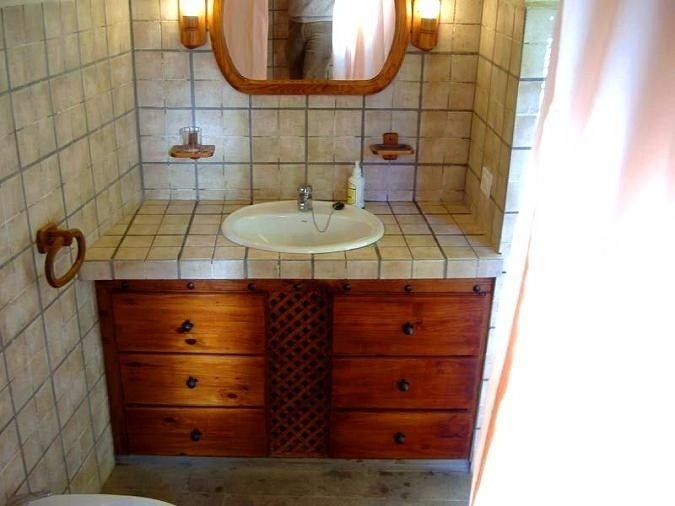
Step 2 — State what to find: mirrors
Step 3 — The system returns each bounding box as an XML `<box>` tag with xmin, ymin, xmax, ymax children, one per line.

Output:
<box><xmin>207</xmin><ymin>0</ymin><xmax>415</xmax><ymax>96</ymax></box>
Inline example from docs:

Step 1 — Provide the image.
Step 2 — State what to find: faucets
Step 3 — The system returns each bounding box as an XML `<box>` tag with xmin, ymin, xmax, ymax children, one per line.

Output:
<box><xmin>295</xmin><ymin>184</ymin><xmax>314</xmax><ymax>211</ymax></box>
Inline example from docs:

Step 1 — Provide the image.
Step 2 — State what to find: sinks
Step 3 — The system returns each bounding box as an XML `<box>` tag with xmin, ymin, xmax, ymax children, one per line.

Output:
<box><xmin>219</xmin><ymin>200</ymin><xmax>385</xmax><ymax>253</ymax></box>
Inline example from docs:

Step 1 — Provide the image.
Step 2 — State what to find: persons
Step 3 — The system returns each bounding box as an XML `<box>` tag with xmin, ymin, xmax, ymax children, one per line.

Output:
<box><xmin>285</xmin><ymin>0</ymin><xmax>335</xmax><ymax>79</ymax></box>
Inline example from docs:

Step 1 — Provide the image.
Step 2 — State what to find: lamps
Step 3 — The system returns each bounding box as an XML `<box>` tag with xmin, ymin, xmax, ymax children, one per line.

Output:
<box><xmin>179</xmin><ymin>0</ymin><xmax>208</xmax><ymax>50</ymax></box>
<box><xmin>409</xmin><ymin>0</ymin><xmax>445</xmax><ymax>53</ymax></box>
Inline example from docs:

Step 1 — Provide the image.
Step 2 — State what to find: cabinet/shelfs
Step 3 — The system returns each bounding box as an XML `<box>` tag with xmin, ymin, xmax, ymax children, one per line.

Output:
<box><xmin>91</xmin><ymin>274</ymin><xmax>274</xmax><ymax>467</ymax></box>
<box><xmin>326</xmin><ymin>272</ymin><xmax>498</xmax><ymax>474</ymax></box>
